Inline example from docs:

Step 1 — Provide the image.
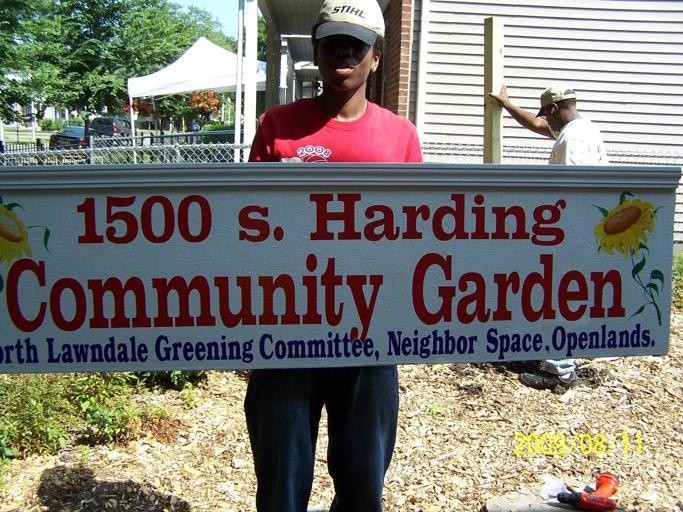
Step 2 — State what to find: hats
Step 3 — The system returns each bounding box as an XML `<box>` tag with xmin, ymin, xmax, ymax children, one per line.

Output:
<box><xmin>535</xmin><ymin>86</ymin><xmax>579</xmax><ymax>117</ymax></box>
<box><xmin>312</xmin><ymin>0</ymin><xmax>386</xmax><ymax>46</ymax></box>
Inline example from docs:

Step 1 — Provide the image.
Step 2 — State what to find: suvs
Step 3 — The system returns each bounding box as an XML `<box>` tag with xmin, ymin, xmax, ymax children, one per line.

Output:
<box><xmin>91</xmin><ymin>117</ymin><xmax>137</xmax><ymax>138</ymax></box>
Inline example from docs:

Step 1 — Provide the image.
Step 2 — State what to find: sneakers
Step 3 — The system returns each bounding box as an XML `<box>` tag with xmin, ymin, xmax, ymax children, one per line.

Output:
<box><xmin>520</xmin><ymin>369</ymin><xmax>569</xmax><ymax>395</ymax></box>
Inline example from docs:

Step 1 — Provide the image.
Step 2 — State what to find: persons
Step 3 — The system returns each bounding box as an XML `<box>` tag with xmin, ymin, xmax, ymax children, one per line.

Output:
<box><xmin>488</xmin><ymin>86</ymin><xmax>608</xmax><ymax>393</ymax></box>
<box><xmin>246</xmin><ymin>0</ymin><xmax>424</xmax><ymax>511</ymax></box>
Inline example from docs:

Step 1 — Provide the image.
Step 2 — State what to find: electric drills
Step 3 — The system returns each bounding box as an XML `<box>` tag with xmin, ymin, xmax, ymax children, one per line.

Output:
<box><xmin>549</xmin><ymin>470</ymin><xmax>620</xmax><ymax>511</ymax></box>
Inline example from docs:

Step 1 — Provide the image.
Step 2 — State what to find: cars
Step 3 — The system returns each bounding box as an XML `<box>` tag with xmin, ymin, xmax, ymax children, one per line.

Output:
<box><xmin>49</xmin><ymin>126</ymin><xmax>98</xmax><ymax>150</ymax></box>
